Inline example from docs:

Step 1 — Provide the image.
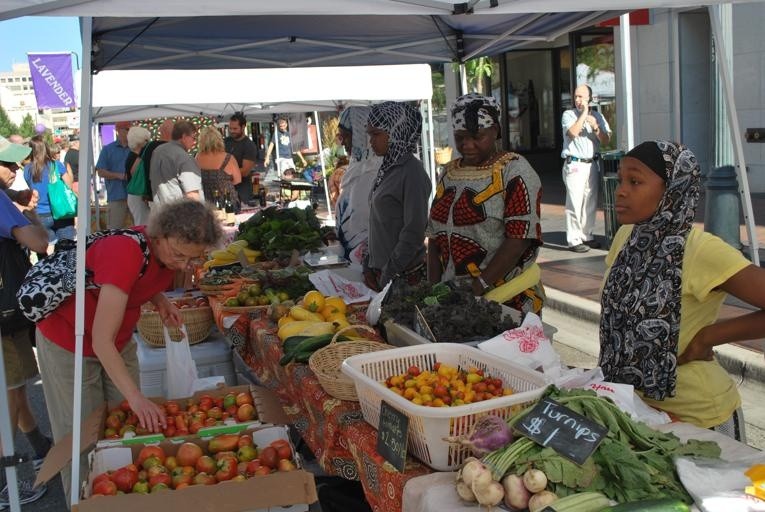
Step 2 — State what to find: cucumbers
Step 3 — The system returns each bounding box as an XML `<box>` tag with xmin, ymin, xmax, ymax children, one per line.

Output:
<box><xmin>278</xmin><ymin>290</ymin><xmax>364</xmax><ymax>367</ymax></box>
<box><xmin>203</xmin><ymin>240</ymin><xmax>261</xmax><ymax>270</ymax></box>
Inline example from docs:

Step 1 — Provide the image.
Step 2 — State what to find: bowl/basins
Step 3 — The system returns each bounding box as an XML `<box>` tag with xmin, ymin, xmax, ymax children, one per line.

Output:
<box><xmin>247</xmin><ymin>199</ymin><xmax>258</xmax><ymax>207</ymax></box>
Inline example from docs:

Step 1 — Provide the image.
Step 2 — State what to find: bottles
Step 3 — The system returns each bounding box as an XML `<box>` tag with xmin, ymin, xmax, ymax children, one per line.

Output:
<box><xmin>213</xmin><ymin>189</ymin><xmax>240</xmax><ymax>213</ymax></box>
<box><xmin>252</xmin><ymin>177</ymin><xmax>259</xmax><ymax>194</ymax></box>
<box><xmin>258</xmin><ymin>188</ymin><xmax>266</xmax><ymax>207</ymax></box>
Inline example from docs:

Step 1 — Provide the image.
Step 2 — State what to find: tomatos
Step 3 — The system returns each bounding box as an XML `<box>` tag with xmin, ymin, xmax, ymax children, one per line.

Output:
<box><xmin>138</xmin><ymin>297</ymin><xmax>209</xmax><ymax>313</ymax></box>
<box><xmin>381</xmin><ymin>363</ymin><xmax>520</xmax><ymax>465</ymax></box>
<box><xmin>92</xmin><ymin>392</ymin><xmax>299</xmax><ymax>497</ymax></box>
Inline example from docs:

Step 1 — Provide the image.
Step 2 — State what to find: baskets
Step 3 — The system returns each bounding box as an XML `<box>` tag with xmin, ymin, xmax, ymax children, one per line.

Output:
<box><xmin>135</xmin><ymin>297</ymin><xmax>214</xmax><ymax>347</ymax></box>
<box><xmin>199</xmin><ymin>273</ymin><xmax>247</xmax><ymax>296</ymax></box>
<box><xmin>222</xmin><ymin>292</ymin><xmax>296</xmax><ymax>314</ymax></box>
<box><xmin>339</xmin><ymin>341</ymin><xmax>553</xmax><ymax>472</ymax></box>
<box><xmin>308</xmin><ymin>324</ymin><xmax>402</xmax><ymax>403</ymax></box>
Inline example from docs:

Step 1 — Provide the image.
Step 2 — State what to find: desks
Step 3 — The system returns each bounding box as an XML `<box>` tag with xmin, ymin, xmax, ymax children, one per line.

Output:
<box><xmin>193</xmin><ymin>263</ymin><xmax>765</xmax><ymax>512</ymax></box>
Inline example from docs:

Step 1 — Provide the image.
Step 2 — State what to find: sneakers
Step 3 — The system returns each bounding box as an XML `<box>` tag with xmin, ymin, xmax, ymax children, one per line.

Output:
<box><xmin>583</xmin><ymin>240</ymin><xmax>600</xmax><ymax>249</ymax></box>
<box><xmin>31</xmin><ymin>436</ymin><xmax>57</xmax><ymax>476</ymax></box>
<box><xmin>0</xmin><ymin>476</ymin><xmax>48</xmax><ymax>510</ymax></box>
<box><xmin>569</xmin><ymin>242</ymin><xmax>591</xmax><ymax>254</ymax></box>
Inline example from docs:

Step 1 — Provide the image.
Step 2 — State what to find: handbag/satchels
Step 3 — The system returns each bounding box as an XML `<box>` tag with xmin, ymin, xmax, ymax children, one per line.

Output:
<box><xmin>0</xmin><ymin>235</ymin><xmax>35</xmax><ymax>338</ymax></box>
<box><xmin>125</xmin><ymin>159</ymin><xmax>146</xmax><ymax>196</ymax></box>
<box><xmin>46</xmin><ymin>160</ymin><xmax>78</xmax><ymax>221</ymax></box>
<box><xmin>16</xmin><ymin>226</ymin><xmax>149</xmax><ymax>323</ymax></box>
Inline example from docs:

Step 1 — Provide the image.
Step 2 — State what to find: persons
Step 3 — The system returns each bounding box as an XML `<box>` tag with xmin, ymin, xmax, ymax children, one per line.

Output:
<box><xmin>560</xmin><ymin>85</ymin><xmax>612</xmax><ymax>253</ymax></box>
<box><xmin>597</xmin><ymin>141</ymin><xmax>764</xmax><ymax>445</ymax></box>
<box><xmin>1</xmin><ymin>101</ymin><xmax>318</xmax><ymax>512</ymax></box>
<box><xmin>329</xmin><ymin>92</ymin><xmax>546</xmax><ymax>320</ymax></box>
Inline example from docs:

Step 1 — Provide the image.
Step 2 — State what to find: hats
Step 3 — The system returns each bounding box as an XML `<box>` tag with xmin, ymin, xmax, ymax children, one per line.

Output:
<box><xmin>68</xmin><ymin>134</ymin><xmax>80</xmax><ymax>141</ymax></box>
<box><xmin>0</xmin><ymin>135</ymin><xmax>32</xmax><ymax>163</ymax></box>
<box><xmin>115</xmin><ymin>122</ymin><xmax>135</xmax><ymax>129</ymax></box>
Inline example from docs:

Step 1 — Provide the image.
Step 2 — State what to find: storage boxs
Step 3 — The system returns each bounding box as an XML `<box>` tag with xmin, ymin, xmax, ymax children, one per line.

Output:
<box><xmin>132</xmin><ymin>325</ymin><xmax>236</xmax><ymax>399</ymax></box>
<box><xmin>32</xmin><ymin>384</ymin><xmax>294</xmax><ymax>490</ymax></box>
<box><xmin>77</xmin><ymin>424</ymin><xmax>319</xmax><ymax>511</ymax></box>
<box><xmin>384</xmin><ymin>297</ymin><xmax>558</xmax><ymax>349</ymax></box>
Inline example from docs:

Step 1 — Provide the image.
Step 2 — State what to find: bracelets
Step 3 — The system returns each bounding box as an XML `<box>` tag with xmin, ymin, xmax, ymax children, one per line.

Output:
<box><xmin>594</xmin><ymin>126</ymin><xmax>601</xmax><ymax>133</ymax></box>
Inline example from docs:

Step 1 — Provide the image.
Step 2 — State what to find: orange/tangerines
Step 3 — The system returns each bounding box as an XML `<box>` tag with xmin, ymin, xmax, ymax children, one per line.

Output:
<box><xmin>224</xmin><ymin>282</ymin><xmax>287</xmax><ymax>308</ymax></box>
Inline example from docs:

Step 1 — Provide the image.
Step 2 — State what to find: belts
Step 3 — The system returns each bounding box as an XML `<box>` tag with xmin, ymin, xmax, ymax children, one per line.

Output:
<box><xmin>568</xmin><ymin>155</ymin><xmax>601</xmax><ymax>163</ymax></box>
